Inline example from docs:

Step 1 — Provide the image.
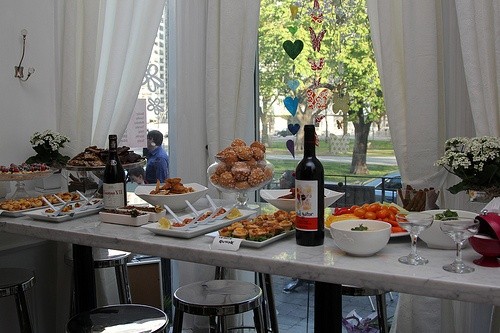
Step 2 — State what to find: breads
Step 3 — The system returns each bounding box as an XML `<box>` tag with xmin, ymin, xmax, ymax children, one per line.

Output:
<box><xmin>67</xmin><ymin>145</ymin><xmax>105</xmax><ymax>167</ymax></box>
<box><xmin>0</xmin><ymin>190</ymin><xmax>81</xmax><ymax>211</ymax></box>
<box><xmin>211</xmin><ymin>138</ymin><xmax>271</xmax><ymax>190</ymax></box>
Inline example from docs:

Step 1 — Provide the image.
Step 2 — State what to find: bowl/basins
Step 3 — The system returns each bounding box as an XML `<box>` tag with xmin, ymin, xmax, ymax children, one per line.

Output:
<box><xmin>405</xmin><ymin>209</ymin><xmax>479</xmax><ymax>249</ymax></box>
<box><xmin>329</xmin><ymin>219</ymin><xmax>392</xmax><ymax>257</ymax></box>
<box><xmin>135</xmin><ymin>182</ymin><xmax>209</xmax><ymax>212</ymax></box>
<box><xmin>259</xmin><ymin>188</ymin><xmax>345</xmax><ymax>213</ymax></box>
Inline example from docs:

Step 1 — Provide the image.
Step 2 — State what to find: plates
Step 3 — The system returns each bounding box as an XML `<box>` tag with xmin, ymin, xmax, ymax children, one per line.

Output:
<box><xmin>140</xmin><ymin>206</ymin><xmax>258</xmax><ymax>239</ymax></box>
<box><xmin>324</xmin><ymin>207</ymin><xmax>410</xmax><ymax>238</ymax></box>
<box><xmin>21</xmin><ymin>198</ymin><xmax>104</xmax><ymax>222</ymax></box>
<box><xmin>0</xmin><ymin>196</ymin><xmax>84</xmax><ymax>218</ymax></box>
<box><xmin>204</xmin><ymin>228</ymin><xmax>296</xmax><ymax>248</ymax></box>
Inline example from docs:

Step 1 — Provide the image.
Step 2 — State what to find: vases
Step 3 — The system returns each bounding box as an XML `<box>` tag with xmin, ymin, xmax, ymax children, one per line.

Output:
<box><xmin>35</xmin><ymin>174</ymin><xmax>63</xmax><ymax>192</ymax></box>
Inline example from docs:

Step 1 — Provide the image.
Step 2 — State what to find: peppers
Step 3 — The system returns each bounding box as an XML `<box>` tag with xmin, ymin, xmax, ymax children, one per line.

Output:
<box><xmin>331</xmin><ymin>207</ymin><xmax>350</xmax><ymax>216</ymax></box>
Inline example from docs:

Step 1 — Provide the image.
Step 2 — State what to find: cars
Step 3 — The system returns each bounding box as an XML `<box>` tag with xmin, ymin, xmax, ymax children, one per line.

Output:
<box><xmin>363</xmin><ymin>171</ymin><xmax>402</xmax><ymax>204</ymax></box>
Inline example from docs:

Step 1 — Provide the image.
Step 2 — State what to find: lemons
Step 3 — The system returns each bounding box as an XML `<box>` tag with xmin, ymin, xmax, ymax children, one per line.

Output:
<box><xmin>227</xmin><ymin>208</ymin><xmax>239</xmax><ymax>220</ymax></box>
<box><xmin>159</xmin><ymin>217</ymin><xmax>170</xmax><ymax>230</ymax></box>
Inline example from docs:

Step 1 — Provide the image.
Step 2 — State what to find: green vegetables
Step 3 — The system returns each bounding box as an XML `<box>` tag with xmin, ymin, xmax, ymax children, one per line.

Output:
<box><xmin>350</xmin><ymin>223</ymin><xmax>368</xmax><ymax>231</ymax></box>
<box><xmin>432</xmin><ymin>209</ymin><xmax>460</xmax><ymax>223</ymax></box>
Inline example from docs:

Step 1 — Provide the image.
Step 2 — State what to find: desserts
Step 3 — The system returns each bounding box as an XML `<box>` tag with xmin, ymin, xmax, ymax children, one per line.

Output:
<box><xmin>218</xmin><ymin>209</ymin><xmax>296</xmax><ymax>241</ymax></box>
<box><xmin>0</xmin><ymin>162</ymin><xmax>52</xmax><ymax>181</ymax></box>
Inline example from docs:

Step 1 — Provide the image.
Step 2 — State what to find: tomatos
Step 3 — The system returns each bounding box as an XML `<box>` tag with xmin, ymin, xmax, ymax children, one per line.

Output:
<box><xmin>350</xmin><ymin>203</ymin><xmax>399</xmax><ymax>224</ymax></box>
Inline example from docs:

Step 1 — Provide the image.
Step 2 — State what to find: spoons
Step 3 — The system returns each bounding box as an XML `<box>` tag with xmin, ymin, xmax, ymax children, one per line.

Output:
<box><xmin>41</xmin><ymin>188</ymin><xmax>103</xmax><ymax>215</ymax></box>
<box><xmin>164</xmin><ymin>195</ymin><xmax>240</xmax><ymax>229</ymax></box>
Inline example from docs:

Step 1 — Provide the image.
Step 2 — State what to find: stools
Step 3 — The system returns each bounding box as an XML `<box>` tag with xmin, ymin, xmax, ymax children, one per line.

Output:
<box><xmin>0</xmin><ymin>248</ymin><xmax>393</xmax><ymax>333</ymax></box>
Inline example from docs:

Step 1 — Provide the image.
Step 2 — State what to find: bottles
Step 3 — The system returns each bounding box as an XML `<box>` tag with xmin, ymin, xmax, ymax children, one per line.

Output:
<box><xmin>294</xmin><ymin>124</ymin><xmax>325</xmax><ymax>246</ymax></box>
<box><xmin>102</xmin><ymin>135</ymin><xmax>127</xmax><ymax>211</ymax></box>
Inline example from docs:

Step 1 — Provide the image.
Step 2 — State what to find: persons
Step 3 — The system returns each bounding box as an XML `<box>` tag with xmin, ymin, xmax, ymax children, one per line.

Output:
<box><xmin>280</xmin><ymin>170</ymin><xmax>295</xmax><ymax>189</ymax></box>
<box><xmin>145</xmin><ymin>130</ymin><xmax>169</xmax><ymax>184</ymax></box>
<box><xmin>129</xmin><ymin>167</ymin><xmax>148</xmax><ymax>184</ymax></box>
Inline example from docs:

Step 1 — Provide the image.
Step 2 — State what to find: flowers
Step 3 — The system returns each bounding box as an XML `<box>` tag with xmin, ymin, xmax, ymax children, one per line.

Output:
<box><xmin>25</xmin><ymin>129</ymin><xmax>71</xmax><ymax>173</ymax></box>
<box><xmin>433</xmin><ymin>136</ymin><xmax>500</xmax><ymax>203</ymax></box>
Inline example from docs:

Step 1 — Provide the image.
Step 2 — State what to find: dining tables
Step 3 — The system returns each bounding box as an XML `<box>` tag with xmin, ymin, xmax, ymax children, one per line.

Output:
<box><xmin>0</xmin><ymin>192</ymin><xmax>500</xmax><ymax>333</ymax></box>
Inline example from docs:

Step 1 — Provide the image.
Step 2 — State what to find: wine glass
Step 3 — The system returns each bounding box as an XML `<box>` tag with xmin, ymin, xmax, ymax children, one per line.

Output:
<box><xmin>440</xmin><ymin>216</ymin><xmax>480</xmax><ymax>274</ymax></box>
<box><xmin>395</xmin><ymin>212</ymin><xmax>434</xmax><ymax>266</ymax></box>
